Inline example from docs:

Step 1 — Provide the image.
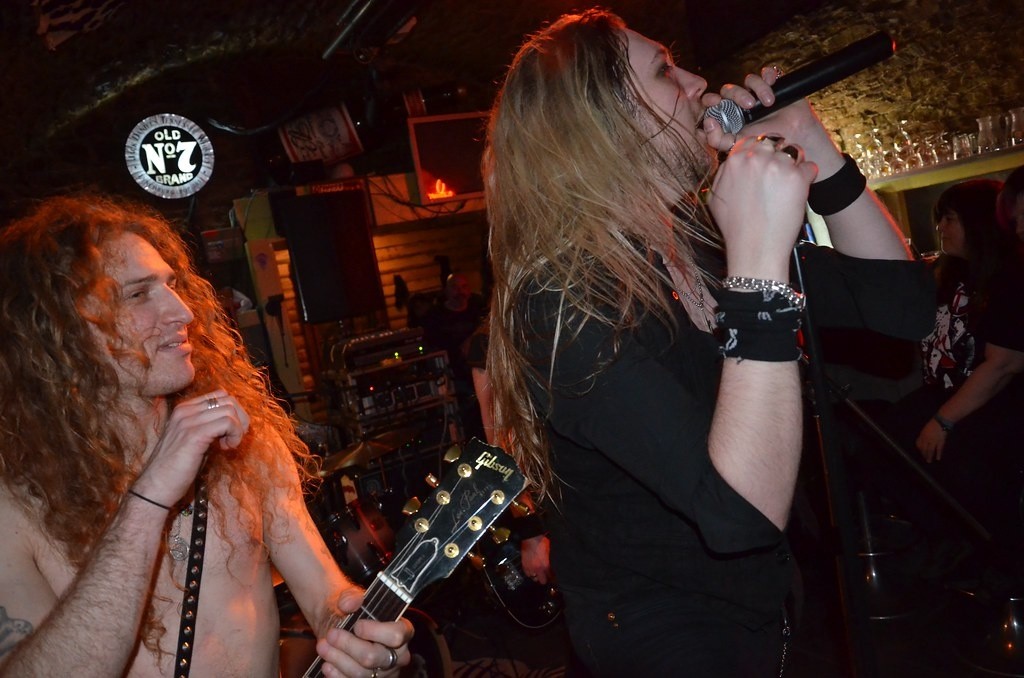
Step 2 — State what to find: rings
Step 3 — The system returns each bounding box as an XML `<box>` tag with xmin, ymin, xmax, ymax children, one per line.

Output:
<box><xmin>377</xmin><ymin>646</ymin><xmax>398</xmax><ymax>671</ymax></box>
<box><xmin>207</xmin><ymin>396</ymin><xmax>218</xmax><ymax>410</ymax></box>
<box><xmin>371</xmin><ymin>665</ymin><xmax>378</xmax><ymax>678</ymax></box>
<box><xmin>780</xmin><ymin>145</ymin><xmax>801</xmax><ymax>166</ymax></box>
<box><xmin>758</xmin><ymin>132</ymin><xmax>785</xmax><ymax>155</ymax></box>
<box><xmin>773</xmin><ymin>65</ymin><xmax>782</xmax><ymax>80</ymax></box>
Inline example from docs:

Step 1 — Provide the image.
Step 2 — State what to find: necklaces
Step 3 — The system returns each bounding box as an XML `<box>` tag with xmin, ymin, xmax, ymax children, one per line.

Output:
<box><xmin>671</xmin><ymin>243</ymin><xmax>715</xmax><ymax>334</ymax></box>
<box><xmin>160</xmin><ymin>508</ymin><xmax>190</xmax><ymax>562</ymax></box>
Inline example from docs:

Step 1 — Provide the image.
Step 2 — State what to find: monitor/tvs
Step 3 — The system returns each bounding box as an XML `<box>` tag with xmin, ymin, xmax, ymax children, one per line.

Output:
<box><xmin>406</xmin><ymin>111</ymin><xmax>491</xmax><ymax>207</ymax></box>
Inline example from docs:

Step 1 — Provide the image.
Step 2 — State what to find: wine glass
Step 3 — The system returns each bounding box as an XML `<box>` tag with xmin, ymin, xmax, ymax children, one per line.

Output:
<box><xmin>843</xmin><ymin>105</ymin><xmax>953</xmax><ymax>182</ymax></box>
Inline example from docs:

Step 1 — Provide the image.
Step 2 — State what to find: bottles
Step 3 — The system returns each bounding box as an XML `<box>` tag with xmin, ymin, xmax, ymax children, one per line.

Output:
<box><xmin>951</xmin><ymin>107</ymin><xmax>1024</xmax><ymax>161</ymax></box>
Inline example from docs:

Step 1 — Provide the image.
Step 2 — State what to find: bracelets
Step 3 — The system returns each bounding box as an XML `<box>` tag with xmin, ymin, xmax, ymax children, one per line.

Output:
<box><xmin>933</xmin><ymin>412</ymin><xmax>955</xmax><ymax>436</ymax></box>
<box><xmin>711</xmin><ymin>290</ymin><xmax>805</xmax><ymax>359</ymax></box>
<box><xmin>514</xmin><ymin>511</ymin><xmax>547</xmax><ymax>541</ymax></box>
<box><xmin>716</xmin><ymin>275</ymin><xmax>808</xmax><ymax>309</ymax></box>
<box><xmin>804</xmin><ymin>151</ymin><xmax>866</xmax><ymax>218</ymax></box>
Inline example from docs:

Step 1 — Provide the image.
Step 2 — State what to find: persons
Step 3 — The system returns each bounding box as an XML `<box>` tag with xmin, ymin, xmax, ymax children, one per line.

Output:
<box><xmin>915</xmin><ymin>176</ymin><xmax>1024</xmax><ymax>524</ymax></box>
<box><xmin>995</xmin><ymin>164</ymin><xmax>1024</xmax><ymax>238</ymax></box>
<box><xmin>126</xmin><ymin>487</ymin><xmax>175</xmax><ymax>514</ymax></box>
<box><xmin>481</xmin><ymin>10</ymin><xmax>985</xmax><ymax>677</ymax></box>
<box><xmin>419</xmin><ymin>268</ymin><xmax>487</xmax><ymax>385</ymax></box>
<box><xmin>0</xmin><ymin>192</ymin><xmax>418</xmax><ymax>678</ymax></box>
<box><xmin>464</xmin><ymin>330</ymin><xmax>557</xmax><ymax>593</ymax></box>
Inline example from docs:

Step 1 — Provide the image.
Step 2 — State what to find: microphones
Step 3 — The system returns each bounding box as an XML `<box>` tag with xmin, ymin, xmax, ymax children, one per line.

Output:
<box><xmin>705</xmin><ymin>30</ymin><xmax>896</xmax><ymax>136</ymax></box>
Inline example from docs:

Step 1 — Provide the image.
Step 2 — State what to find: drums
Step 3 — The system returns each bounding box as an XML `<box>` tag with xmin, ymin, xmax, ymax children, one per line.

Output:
<box><xmin>317</xmin><ymin>498</ymin><xmax>405</xmax><ymax>582</ymax></box>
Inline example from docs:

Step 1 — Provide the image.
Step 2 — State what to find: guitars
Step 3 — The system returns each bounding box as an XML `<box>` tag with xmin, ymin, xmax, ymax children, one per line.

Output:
<box><xmin>474</xmin><ymin>505</ymin><xmax>566</xmax><ymax>629</ymax></box>
<box><xmin>294</xmin><ymin>434</ymin><xmax>536</xmax><ymax>678</ymax></box>
